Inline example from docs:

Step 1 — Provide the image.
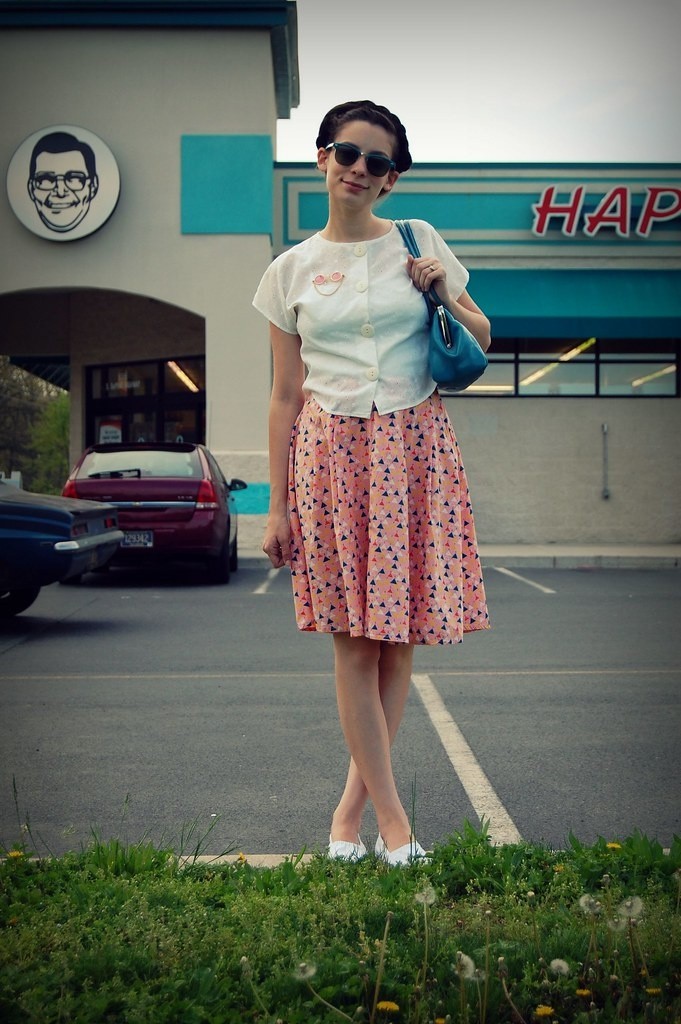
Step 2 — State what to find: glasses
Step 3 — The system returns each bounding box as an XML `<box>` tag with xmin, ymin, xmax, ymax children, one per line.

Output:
<box><xmin>325</xmin><ymin>142</ymin><xmax>396</xmax><ymax>178</ymax></box>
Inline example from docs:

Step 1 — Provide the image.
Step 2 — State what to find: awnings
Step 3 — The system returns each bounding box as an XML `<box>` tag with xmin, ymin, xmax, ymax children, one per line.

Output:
<box><xmin>454</xmin><ymin>270</ymin><xmax>681</xmax><ymax>339</ymax></box>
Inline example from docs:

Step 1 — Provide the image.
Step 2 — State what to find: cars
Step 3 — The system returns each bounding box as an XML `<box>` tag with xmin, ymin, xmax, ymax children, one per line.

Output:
<box><xmin>60</xmin><ymin>440</ymin><xmax>247</xmax><ymax>584</ymax></box>
<box><xmin>0</xmin><ymin>479</ymin><xmax>125</xmax><ymax>620</ymax></box>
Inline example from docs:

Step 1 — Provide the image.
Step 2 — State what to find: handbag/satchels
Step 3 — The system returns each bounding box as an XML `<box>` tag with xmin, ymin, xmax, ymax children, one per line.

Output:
<box><xmin>395</xmin><ymin>219</ymin><xmax>488</xmax><ymax>392</ymax></box>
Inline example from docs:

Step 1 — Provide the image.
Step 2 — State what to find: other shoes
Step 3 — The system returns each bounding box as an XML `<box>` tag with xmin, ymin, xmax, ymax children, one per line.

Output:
<box><xmin>374</xmin><ymin>832</ymin><xmax>433</xmax><ymax>868</ymax></box>
<box><xmin>329</xmin><ymin>832</ymin><xmax>368</xmax><ymax>866</ymax></box>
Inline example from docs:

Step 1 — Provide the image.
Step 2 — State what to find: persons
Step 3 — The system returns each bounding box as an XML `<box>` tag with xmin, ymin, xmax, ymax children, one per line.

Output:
<box><xmin>251</xmin><ymin>99</ymin><xmax>490</xmax><ymax>867</ymax></box>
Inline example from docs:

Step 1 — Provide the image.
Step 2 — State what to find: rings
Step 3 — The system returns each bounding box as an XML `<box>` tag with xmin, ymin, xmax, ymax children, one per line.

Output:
<box><xmin>430</xmin><ymin>265</ymin><xmax>435</xmax><ymax>271</ymax></box>
